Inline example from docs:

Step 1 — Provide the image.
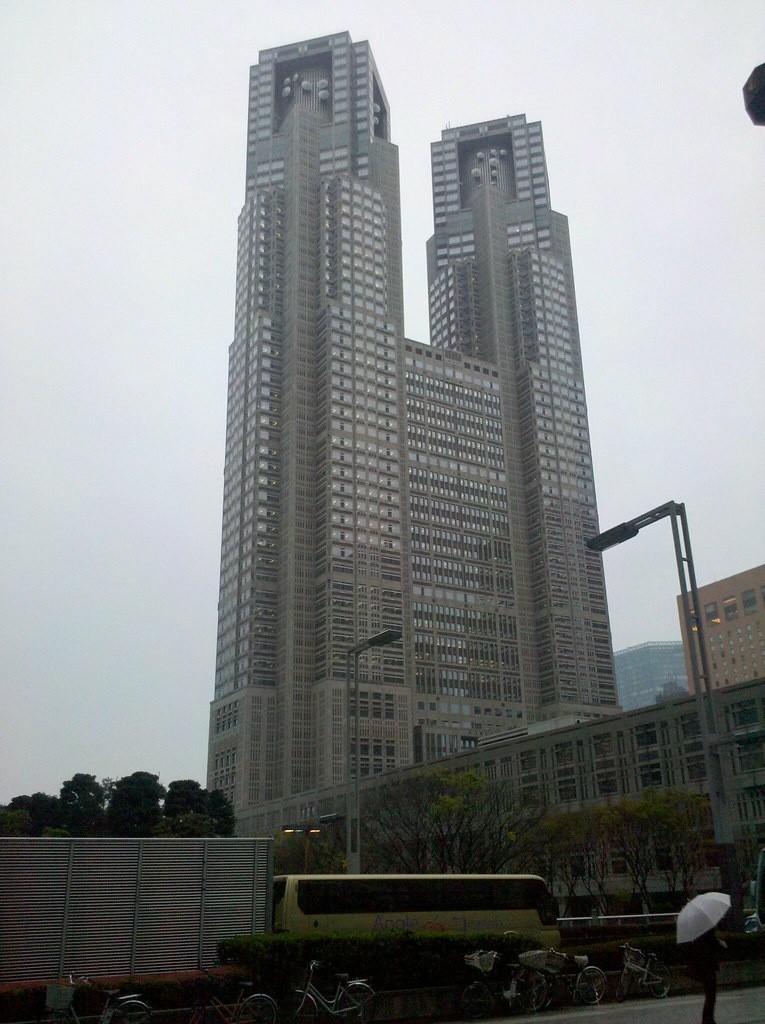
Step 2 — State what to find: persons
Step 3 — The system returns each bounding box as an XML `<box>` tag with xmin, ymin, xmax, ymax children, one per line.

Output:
<box><xmin>683</xmin><ymin>928</ymin><xmax>727</xmax><ymax>1024</ymax></box>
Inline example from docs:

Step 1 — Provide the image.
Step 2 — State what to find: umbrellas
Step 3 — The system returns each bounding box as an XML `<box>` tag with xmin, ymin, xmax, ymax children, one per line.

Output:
<box><xmin>676</xmin><ymin>892</ymin><xmax>731</xmax><ymax>944</ymax></box>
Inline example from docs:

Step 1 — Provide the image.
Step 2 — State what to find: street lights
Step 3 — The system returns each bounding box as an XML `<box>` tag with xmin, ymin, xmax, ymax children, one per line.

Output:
<box><xmin>587</xmin><ymin>501</ymin><xmax>744</xmax><ymax>938</ymax></box>
<box><xmin>282</xmin><ymin>823</ymin><xmax>320</xmax><ymax>873</ymax></box>
<box><xmin>345</xmin><ymin>630</ymin><xmax>402</xmax><ymax>875</ymax></box>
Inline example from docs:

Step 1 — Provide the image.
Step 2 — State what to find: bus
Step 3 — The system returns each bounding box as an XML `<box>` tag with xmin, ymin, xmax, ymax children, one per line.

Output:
<box><xmin>273</xmin><ymin>872</ymin><xmax>561</xmax><ymax>948</ymax></box>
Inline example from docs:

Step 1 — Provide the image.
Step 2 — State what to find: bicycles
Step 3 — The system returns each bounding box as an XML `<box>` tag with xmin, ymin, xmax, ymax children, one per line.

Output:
<box><xmin>46</xmin><ymin>972</ymin><xmax>153</xmax><ymax>1024</ymax></box>
<box><xmin>186</xmin><ymin>968</ymin><xmax>278</xmax><ymax>1024</ymax></box>
<box><xmin>460</xmin><ymin>950</ymin><xmax>548</xmax><ymax>1019</ymax></box>
<box><xmin>527</xmin><ymin>948</ymin><xmax>607</xmax><ymax>1006</ymax></box>
<box><xmin>278</xmin><ymin>960</ymin><xmax>377</xmax><ymax>1024</ymax></box>
<box><xmin>617</xmin><ymin>943</ymin><xmax>671</xmax><ymax>1003</ymax></box>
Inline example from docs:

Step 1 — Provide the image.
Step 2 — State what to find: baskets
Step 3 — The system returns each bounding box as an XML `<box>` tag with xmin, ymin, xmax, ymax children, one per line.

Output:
<box><xmin>623</xmin><ymin>952</ymin><xmax>644</xmax><ymax>971</ymax></box>
<box><xmin>291</xmin><ymin>974</ymin><xmax>309</xmax><ymax>990</ymax></box>
<box><xmin>46</xmin><ymin>983</ymin><xmax>76</xmax><ymax>1009</ymax></box>
<box><xmin>545</xmin><ymin>955</ymin><xmax>563</xmax><ymax>973</ymax></box>
